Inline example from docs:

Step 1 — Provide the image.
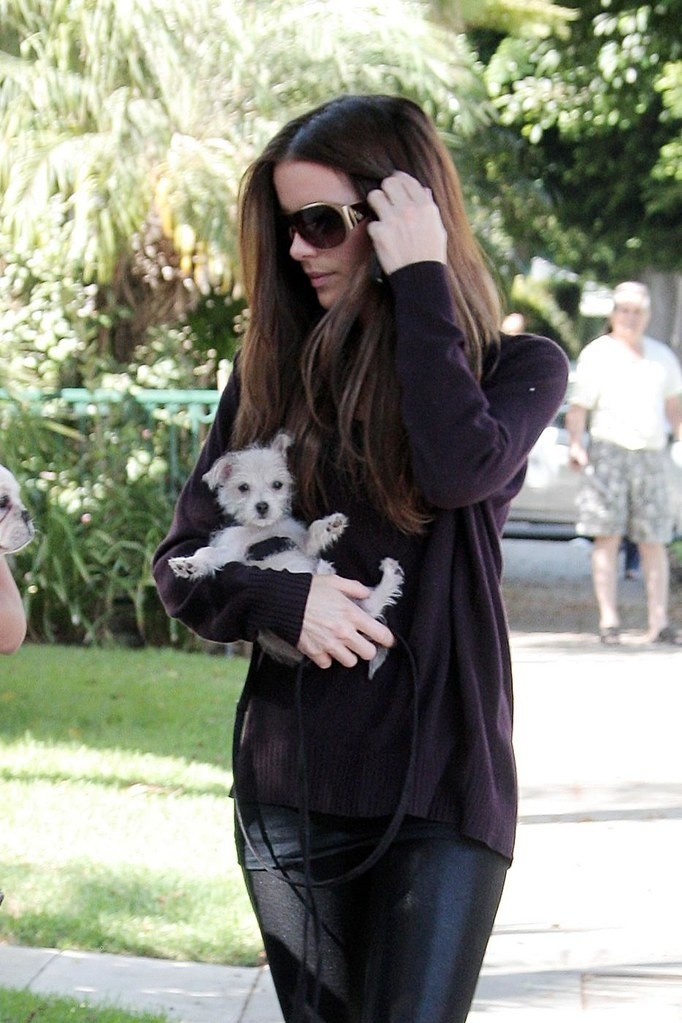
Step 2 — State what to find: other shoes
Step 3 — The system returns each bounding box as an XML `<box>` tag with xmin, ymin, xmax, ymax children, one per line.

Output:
<box><xmin>599</xmin><ymin>626</ymin><xmax>621</xmax><ymax>647</ymax></box>
<box><xmin>649</xmin><ymin>624</ymin><xmax>682</xmax><ymax>645</ymax></box>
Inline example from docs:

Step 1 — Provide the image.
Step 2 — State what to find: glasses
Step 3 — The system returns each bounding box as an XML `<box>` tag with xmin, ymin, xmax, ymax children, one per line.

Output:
<box><xmin>279</xmin><ymin>199</ymin><xmax>379</xmax><ymax>249</ymax></box>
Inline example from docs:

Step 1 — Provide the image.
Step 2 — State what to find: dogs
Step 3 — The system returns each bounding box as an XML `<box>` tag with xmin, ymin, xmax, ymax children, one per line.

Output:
<box><xmin>167</xmin><ymin>431</ymin><xmax>404</xmax><ymax>666</ymax></box>
<box><xmin>0</xmin><ymin>462</ymin><xmax>37</xmax><ymax>554</ymax></box>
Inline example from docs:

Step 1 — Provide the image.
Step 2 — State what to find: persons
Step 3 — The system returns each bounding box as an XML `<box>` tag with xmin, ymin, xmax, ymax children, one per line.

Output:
<box><xmin>0</xmin><ymin>553</ymin><xmax>27</xmax><ymax>656</ymax></box>
<box><xmin>566</xmin><ymin>281</ymin><xmax>682</xmax><ymax>647</ymax></box>
<box><xmin>151</xmin><ymin>95</ymin><xmax>568</xmax><ymax>1023</ymax></box>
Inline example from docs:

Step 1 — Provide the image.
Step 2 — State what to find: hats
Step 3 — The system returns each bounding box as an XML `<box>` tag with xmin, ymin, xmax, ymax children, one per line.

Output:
<box><xmin>613</xmin><ymin>280</ymin><xmax>651</xmax><ymax>308</ymax></box>
